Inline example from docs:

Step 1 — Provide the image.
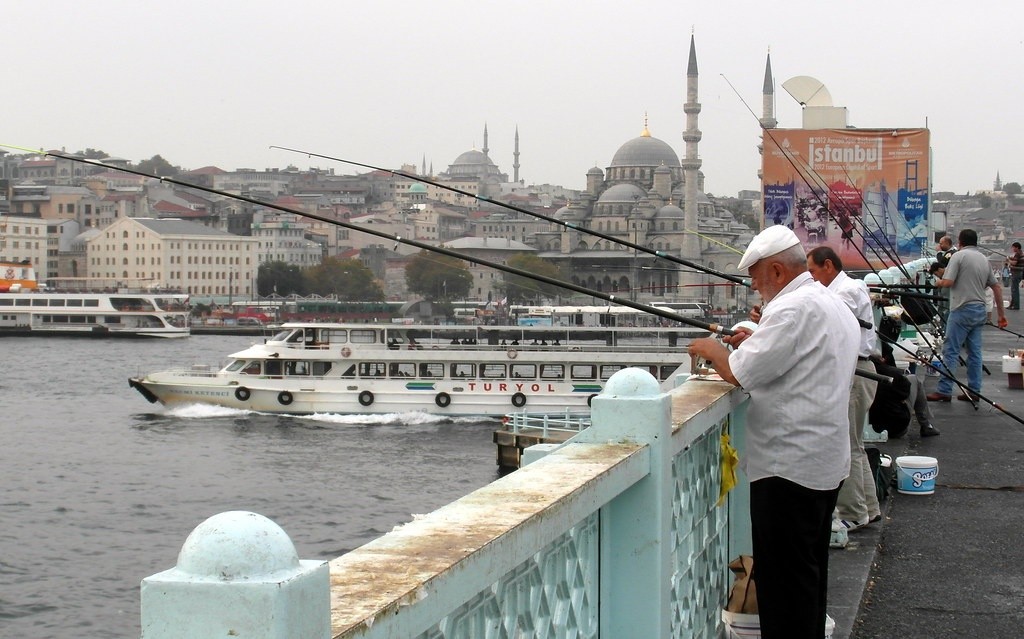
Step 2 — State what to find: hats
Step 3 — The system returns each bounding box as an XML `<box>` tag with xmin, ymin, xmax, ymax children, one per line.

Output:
<box><xmin>736</xmin><ymin>226</ymin><xmax>800</xmax><ymax>272</ymax></box>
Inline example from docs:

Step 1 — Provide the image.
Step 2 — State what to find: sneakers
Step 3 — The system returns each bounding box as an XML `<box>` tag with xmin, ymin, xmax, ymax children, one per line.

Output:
<box><xmin>830</xmin><ymin>510</ymin><xmax>882</xmax><ymax>532</ymax></box>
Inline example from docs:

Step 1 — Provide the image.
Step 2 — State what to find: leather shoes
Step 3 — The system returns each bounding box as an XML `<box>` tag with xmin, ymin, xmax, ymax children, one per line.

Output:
<box><xmin>958</xmin><ymin>392</ymin><xmax>979</xmax><ymax>401</ymax></box>
<box><xmin>927</xmin><ymin>391</ymin><xmax>951</xmax><ymax>402</ymax></box>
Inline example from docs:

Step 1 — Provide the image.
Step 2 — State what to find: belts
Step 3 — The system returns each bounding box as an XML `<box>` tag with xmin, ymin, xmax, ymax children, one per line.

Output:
<box><xmin>857</xmin><ymin>354</ymin><xmax>871</xmax><ymax>362</ymax></box>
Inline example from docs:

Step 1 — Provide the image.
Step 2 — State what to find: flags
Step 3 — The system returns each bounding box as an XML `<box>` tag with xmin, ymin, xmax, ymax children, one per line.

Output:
<box><xmin>500</xmin><ymin>298</ymin><xmax>507</xmax><ymax>305</ymax></box>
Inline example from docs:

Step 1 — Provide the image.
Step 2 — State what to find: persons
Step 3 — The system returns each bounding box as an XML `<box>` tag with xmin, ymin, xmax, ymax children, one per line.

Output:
<box><xmin>501</xmin><ymin>340</ymin><xmax>507</xmax><ymax>351</ymax></box>
<box><xmin>869</xmin><ymin>316</ymin><xmax>941</xmax><ymax>440</ymax></box>
<box><xmin>836</xmin><ymin>211</ymin><xmax>853</xmax><ymax>250</ymax></box>
<box><xmin>451</xmin><ymin>338</ymin><xmax>476</xmax><ymax>351</ymax></box>
<box><xmin>926</xmin><ymin>229</ymin><xmax>1008</xmax><ymax>403</ymax></box>
<box><xmin>428</xmin><ymin>371</ymin><xmax>432</xmax><ymax>376</ymax></box>
<box><xmin>500</xmin><ymin>373</ymin><xmax>504</xmax><ymax>378</ymax></box>
<box><xmin>510</xmin><ymin>339</ymin><xmax>519</xmax><ymax>351</ymax></box>
<box><xmin>530</xmin><ymin>339</ymin><xmax>561</xmax><ymax>352</ymax></box>
<box><xmin>809</xmin><ymin>199</ymin><xmax>826</xmax><ymax>239</ymax></box>
<box><xmin>391</xmin><ymin>339</ymin><xmax>400</xmax><ymax>349</ymax></box>
<box><xmin>399</xmin><ymin>371</ymin><xmax>410</xmax><ymax>377</ymax></box>
<box><xmin>1007</xmin><ymin>242</ymin><xmax>1024</xmax><ymax>310</ymax></box>
<box><xmin>460</xmin><ymin>371</ymin><xmax>464</xmax><ymax>376</ymax></box>
<box><xmin>1002</xmin><ymin>263</ymin><xmax>1009</xmax><ymax>287</ymax></box>
<box><xmin>515</xmin><ymin>372</ymin><xmax>521</xmax><ymax>378</ymax></box>
<box><xmin>751</xmin><ymin>247</ymin><xmax>882</xmax><ymax>532</ymax></box>
<box><xmin>689</xmin><ymin>225</ymin><xmax>863</xmax><ymax>639</ymax></box>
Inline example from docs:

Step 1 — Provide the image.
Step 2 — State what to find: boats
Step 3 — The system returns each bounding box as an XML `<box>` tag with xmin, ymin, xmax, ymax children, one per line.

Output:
<box><xmin>129</xmin><ymin>322</ymin><xmax>712</xmax><ymax>417</ymax></box>
<box><xmin>0</xmin><ymin>277</ymin><xmax>191</xmax><ymax>338</ymax></box>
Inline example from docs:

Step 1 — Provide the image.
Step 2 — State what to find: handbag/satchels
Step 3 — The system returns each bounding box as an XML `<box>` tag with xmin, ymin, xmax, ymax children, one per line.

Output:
<box><xmin>726</xmin><ymin>554</ymin><xmax>758</xmax><ymax>614</ymax></box>
<box><xmin>866</xmin><ymin>448</ymin><xmax>889</xmax><ymax>503</ymax></box>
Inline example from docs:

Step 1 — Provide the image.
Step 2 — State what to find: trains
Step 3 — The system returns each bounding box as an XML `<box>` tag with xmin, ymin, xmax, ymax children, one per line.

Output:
<box><xmin>232</xmin><ymin>300</ymin><xmax>712</xmax><ymax>325</ymax></box>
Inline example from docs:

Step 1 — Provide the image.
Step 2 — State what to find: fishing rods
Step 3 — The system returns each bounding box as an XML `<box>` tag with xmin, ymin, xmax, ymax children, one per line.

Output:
<box><xmin>875</xmin><ymin>329</ymin><xmax>1024</xmax><ymax>425</ymax></box>
<box><xmin>266</xmin><ymin>143</ymin><xmax>878</xmax><ymax>332</ymax></box>
<box><xmin>555</xmin><ymin>264</ymin><xmax>950</xmax><ymax>302</ymax></box>
<box><xmin>43</xmin><ymin>150</ymin><xmax>898</xmax><ymax>386</ymax></box>
<box><xmin>918</xmin><ymin>221</ymin><xmax>1007</xmax><ymax>258</ymax></box>
<box><xmin>718</xmin><ymin>72</ymin><xmax>1024</xmax><ymax>411</ymax></box>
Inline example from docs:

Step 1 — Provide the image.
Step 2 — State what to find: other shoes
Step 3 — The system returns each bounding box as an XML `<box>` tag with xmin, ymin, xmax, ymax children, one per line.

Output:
<box><xmin>921</xmin><ymin>424</ymin><xmax>941</xmax><ymax>437</ymax></box>
<box><xmin>1006</xmin><ymin>304</ymin><xmax>1021</xmax><ymax>310</ymax></box>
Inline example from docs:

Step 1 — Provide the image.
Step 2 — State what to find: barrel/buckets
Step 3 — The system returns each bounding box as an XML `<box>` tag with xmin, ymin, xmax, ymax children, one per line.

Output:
<box><xmin>895</xmin><ymin>455</ymin><xmax>939</xmax><ymax>495</ymax></box>
<box><xmin>926</xmin><ymin>354</ymin><xmax>943</xmax><ymax>377</ymax></box>
<box><xmin>721</xmin><ymin>607</ymin><xmax>761</xmax><ymax>639</ymax></box>
<box><xmin>825</xmin><ymin>614</ymin><xmax>835</xmax><ymax>639</ymax></box>
